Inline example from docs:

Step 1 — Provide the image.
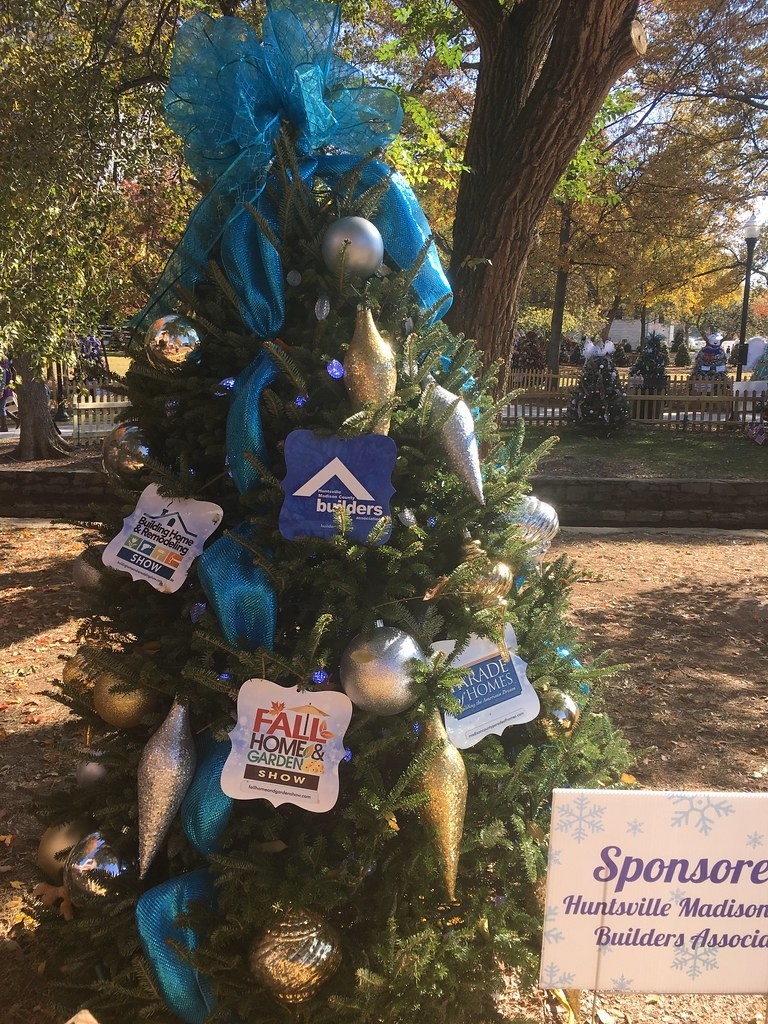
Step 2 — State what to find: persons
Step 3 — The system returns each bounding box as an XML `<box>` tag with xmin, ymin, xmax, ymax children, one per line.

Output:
<box><xmin>0</xmin><ymin>350</ymin><xmax>20</xmax><ymax>432</ymax></box>
<box><xmin>81</xmin><ymin>331</ymin><xmax>102</xmax><ymax>353</ymax></box>
<box><xmin>726</xmin><ymin>346</ymin><xmax>730</xmax><ymax>357</ymax></box>
<box><xmin>622</xmin><ymin>339</ymin><xmax>631</xmax><ymax>353</ymax></box>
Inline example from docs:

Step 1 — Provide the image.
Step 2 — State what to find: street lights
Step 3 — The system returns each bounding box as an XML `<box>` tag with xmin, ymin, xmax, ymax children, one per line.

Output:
<box><xmin>736</xmin><ymin>210</ymin><xmax>761</xmax><ymax>381</ymax></box>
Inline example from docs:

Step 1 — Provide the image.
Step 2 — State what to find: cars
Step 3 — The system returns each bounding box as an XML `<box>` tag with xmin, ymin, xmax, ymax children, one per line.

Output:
<box><xmin>692</xmin><ymin>337</ymin><xmax>707</xmax><ymax>351</ymax></box>
